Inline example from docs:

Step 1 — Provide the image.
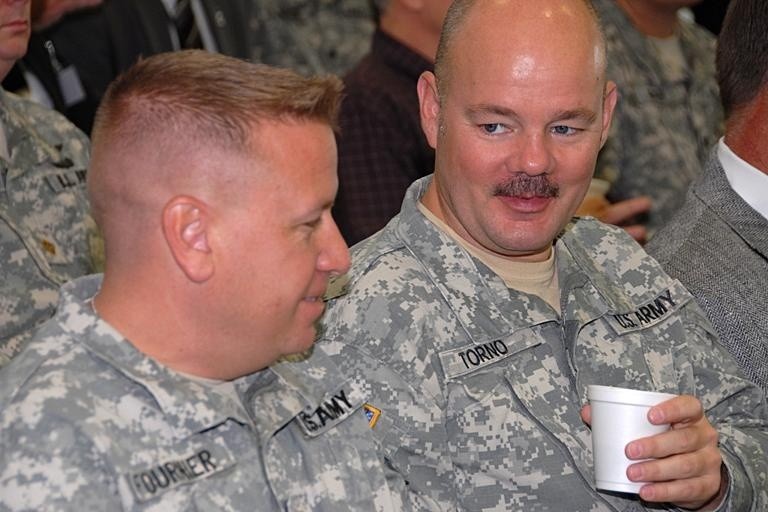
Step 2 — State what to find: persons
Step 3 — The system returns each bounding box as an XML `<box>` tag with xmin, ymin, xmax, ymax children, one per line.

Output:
<box><xmin>1</xmin><ymin>50</ymin><xmax>413</xmax><ymax>512</ymax></box>
<box><xmin>318</xmin><ymin>1</ymin><xmax>768</xmax><ymax>512</ymax></box>
<box><xmin>0</xmin><ymin>0</ymin><xmax>768</xmax><ymax>397</ymax></box>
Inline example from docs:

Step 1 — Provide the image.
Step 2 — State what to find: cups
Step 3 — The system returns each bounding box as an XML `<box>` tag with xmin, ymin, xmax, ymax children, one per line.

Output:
<box><xmin>586</xmin><ymin>384</ymin><xmax>681</xmax><ymax>495</ymax></box>
